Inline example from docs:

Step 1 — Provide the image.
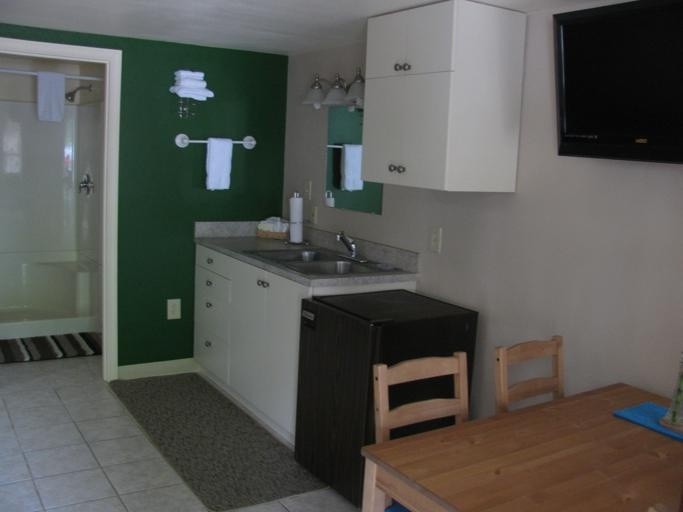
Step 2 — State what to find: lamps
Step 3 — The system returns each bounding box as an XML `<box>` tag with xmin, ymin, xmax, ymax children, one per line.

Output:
<box><xmin>344</xmin><ymin>66</ymin><xmax>365</xmax><ymax>103</ymax></box>
<box><xmin>301</xmin><ymin>74</ymin><xmax>331</xmax><ymax>110</ymax></box>
<box><xmin>322</xmin><ymin>73</ymin><xmax>348</xmax><ymax>105</ymax></box>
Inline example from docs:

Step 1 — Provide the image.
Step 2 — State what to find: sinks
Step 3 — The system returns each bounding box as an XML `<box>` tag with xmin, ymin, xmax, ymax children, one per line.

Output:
<box><xmin>284</xmin><ymin>259</ymin><xmax>383</xmax><ymax>275</ymax></box>
<box><xmin>242</xmin><ymin>247</ymin><xmax>353</xmax><ymax>262</ymax></box>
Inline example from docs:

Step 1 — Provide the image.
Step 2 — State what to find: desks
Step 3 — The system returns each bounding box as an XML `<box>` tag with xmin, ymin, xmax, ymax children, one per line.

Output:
<box><xmin>361</xmin><ymin>383</ymin><xmax>683</xmax><ymax>512</ymax></box>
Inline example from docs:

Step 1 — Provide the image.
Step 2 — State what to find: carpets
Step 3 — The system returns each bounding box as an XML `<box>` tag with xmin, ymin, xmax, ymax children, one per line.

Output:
<box><xmin>0</xmin><ymin>332</ymin><xmax>102</xmax><ymax>364</ymax></box>
<box><xmin>108</xmin><ymin>372</ymin><xmax>331</xmax><ymax>512</ymax></box>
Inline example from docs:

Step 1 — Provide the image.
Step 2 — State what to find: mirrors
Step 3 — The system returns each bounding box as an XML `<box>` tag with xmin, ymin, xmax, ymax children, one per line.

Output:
<box><xmin>325</xmin><ymin>105</ymin><xmax>383</xmax><ymax>216</ymax></box>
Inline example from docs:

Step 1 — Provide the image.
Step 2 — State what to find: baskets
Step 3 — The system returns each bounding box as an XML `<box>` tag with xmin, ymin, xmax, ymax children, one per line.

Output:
<box><xmin>256</xmin><ymin>231</ymin><xmax>288</xmax><ymax>240</ymax></box>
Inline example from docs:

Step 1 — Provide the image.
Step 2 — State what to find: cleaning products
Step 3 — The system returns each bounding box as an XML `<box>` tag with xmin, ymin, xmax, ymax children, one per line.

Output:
<box><xmin>326</xmin><ymin>190</ymin><xmax>336</xmax><ymax>207</ymax></box>
<box><xmin>287</xmin><ymin>189</ymin><xmax>306</xmax><ymax>243</ymax></box>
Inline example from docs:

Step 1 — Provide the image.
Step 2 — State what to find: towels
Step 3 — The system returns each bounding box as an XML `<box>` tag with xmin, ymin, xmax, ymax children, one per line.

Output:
<box><xmin>340</xmin><ymin>144</ymin><xmax>364</xmax><ymax>192</ymax></box>
<box><xmin>170</xmin><ymin>69</ymin><xmax>215</xmax><ymax>101</ymax></box>
<box><xmin>36</xmin><ymin>71</ymin><xmax>65</xmax><ymax>122</ymax></box>
<box><xmin>206</xmin><ymin>136</ymin><xmax>233</xmax><ymax>190</ymax></box>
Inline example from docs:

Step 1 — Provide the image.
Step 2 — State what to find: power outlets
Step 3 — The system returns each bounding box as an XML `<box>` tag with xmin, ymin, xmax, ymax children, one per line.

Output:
<box><xmin>167</xmin><ymin>298</ymin><xmax>183</xmax><ymax>321</ymax></box>
<box><xmin>430</xmin><ymin>226</ymin><xmax>454</xmax><ymax>257</ymax></box>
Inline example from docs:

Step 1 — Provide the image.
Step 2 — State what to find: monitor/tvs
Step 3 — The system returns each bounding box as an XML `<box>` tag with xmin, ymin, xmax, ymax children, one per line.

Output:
<box><xmin>551</xmin><ymin>1</ymin><xmax>683</xmax><ymax>166</ymax></box>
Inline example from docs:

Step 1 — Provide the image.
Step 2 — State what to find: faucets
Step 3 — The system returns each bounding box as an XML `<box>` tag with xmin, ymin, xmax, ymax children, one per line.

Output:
<box><xmin>335</xmin><ymin>226</ymin><xmax>360</xmax><ymax>258</ymax></box>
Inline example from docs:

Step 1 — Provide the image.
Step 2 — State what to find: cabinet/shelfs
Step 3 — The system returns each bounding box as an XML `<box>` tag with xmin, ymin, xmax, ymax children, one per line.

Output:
<box><xmin>294</xmin><ymin>288</ymin><xmax>479</xmax><ymax>509</ymax></box>
<box><xmin>191</xmin><ymin>243</ymin><xmax>231</xmax><ymax>395</ymax></box>
<box><xmin>224</xmin><ymin>258</ymin><xmax>415</xmax><ymax>452</ymax></box>
<box><xmin>361</xmin><ymin>0</ymin><xmax>527</xmax><ymax>191</ymax></box>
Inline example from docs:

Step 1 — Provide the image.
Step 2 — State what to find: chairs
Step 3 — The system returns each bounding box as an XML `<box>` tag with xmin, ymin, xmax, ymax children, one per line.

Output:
<box><xmin>372</xmin><ymin>352</ymin><xmax>469</xmax><ymax>445</ymax></box>
<box><xmin>493</xmin><ymin>335</ymin><xmax>563</xmax><ymax>413</ymax></box>
